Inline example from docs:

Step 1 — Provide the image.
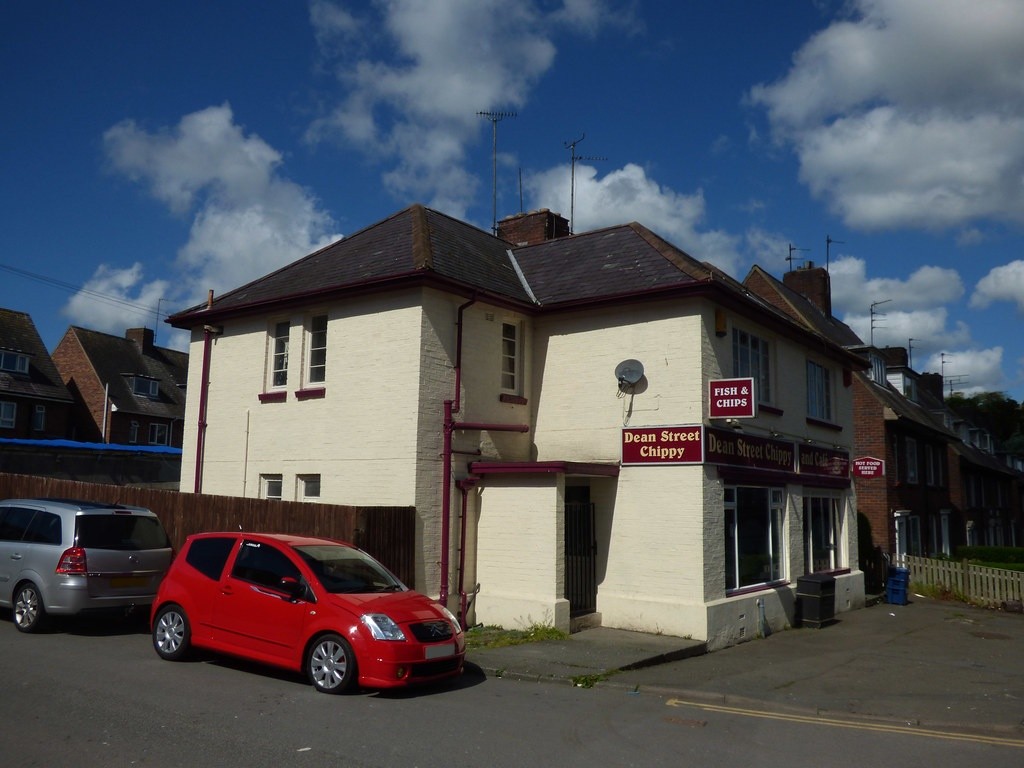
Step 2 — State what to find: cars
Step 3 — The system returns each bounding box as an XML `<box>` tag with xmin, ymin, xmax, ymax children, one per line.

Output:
<box><xmin>0</xmin><ymin>497</ymin><xmax>175</xmax><ymax>634</ymax></box>
<box><xmin>149</xmin><ymin>531</ymin><xmax>467</xmax><ymax>695</ymax></box>
